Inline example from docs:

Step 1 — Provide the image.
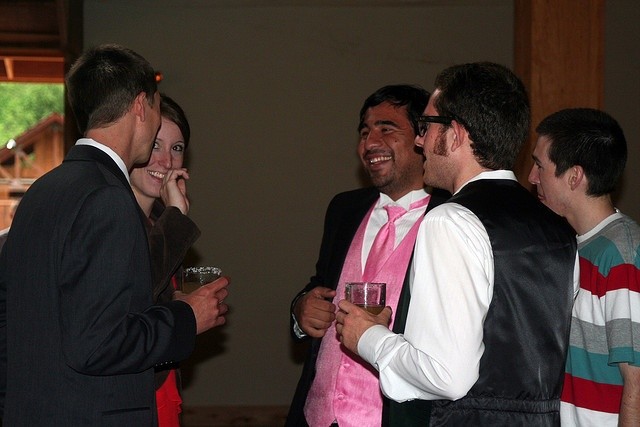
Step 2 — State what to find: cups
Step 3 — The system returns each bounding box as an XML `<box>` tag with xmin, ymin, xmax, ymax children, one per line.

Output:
<box><xmin>182</xmin><ymin>265</ymin><xmax>222</xmax><ymax>293</ymax></box>
<box><xmin>345</xmin><ymin>283</ymin><xmax>386</xmax><ymax>314</ymax></box>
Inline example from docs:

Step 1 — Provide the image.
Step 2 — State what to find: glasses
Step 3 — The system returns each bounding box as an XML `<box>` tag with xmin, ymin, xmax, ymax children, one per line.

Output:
<box><xmin>415</xmin><ymin>115</ymin><xmax>454</xmax><ymax>137</ymax></box>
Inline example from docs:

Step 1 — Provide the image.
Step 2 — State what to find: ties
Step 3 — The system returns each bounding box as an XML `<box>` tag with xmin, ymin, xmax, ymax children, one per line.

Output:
<box><xmin>364</xmin><ymin>196</ymin><xmax>429</xmax><ymax>282</ymax></box>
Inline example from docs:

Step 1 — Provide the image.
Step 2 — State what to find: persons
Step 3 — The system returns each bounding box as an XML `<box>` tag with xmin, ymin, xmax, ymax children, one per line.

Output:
<box><xmin>335</xmin><ymin>61</ymin><xmax>579</xmax><ymax>427</ymax></box>
<box><xmin>127</xmin><ymin>94</ymin><xmax>205</xmax><ymax>427</ymax></box>
<box><xmin>0</xmin><ymin>44</ymin><xmax>231</xmax><ymax>426</ymax></box>
<box><xmin>528</xmin><ymin>107</ymin><xmax>640</xmax><ymax>427</ymax></box>
<box><xmin>286</xmin><ymin>85</ymin><xmax>452</xmax><ymax>427</ymax></box>
